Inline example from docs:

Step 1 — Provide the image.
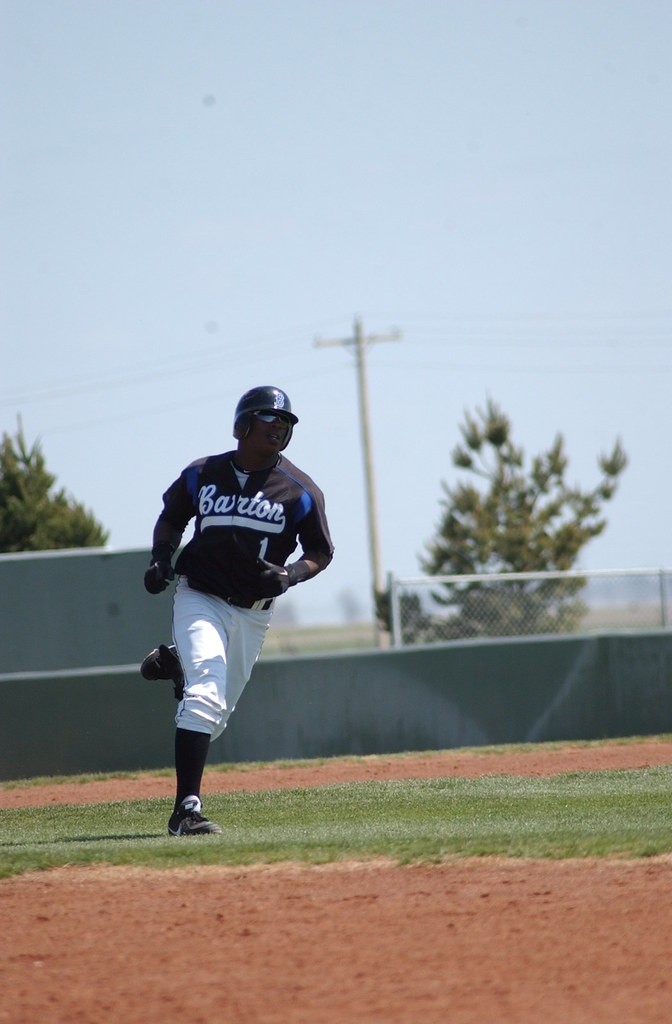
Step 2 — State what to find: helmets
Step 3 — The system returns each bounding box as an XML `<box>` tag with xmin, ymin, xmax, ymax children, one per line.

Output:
<box><xmin>233</xmin><ymin>386</ymin><xmax>297</xmax><ymax>452</ymax></box>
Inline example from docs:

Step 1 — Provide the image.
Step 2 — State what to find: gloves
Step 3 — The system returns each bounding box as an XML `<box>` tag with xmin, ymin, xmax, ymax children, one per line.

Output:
<box><xmin>252</xmin><ymin>559</ymin><xmax>310</xmax><ymax>598</ymax></box>
<box><xmin>145</xmin><ymin>559</ymin><xmax>175</xmax><ymax>594</ymax></box>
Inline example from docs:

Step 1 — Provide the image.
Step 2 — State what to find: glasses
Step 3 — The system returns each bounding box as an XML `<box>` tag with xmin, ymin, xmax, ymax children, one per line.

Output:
<box><xmin>253</xmin><ymin>411</ymin><xmax>289</xmax><ymax>424</ymax></box>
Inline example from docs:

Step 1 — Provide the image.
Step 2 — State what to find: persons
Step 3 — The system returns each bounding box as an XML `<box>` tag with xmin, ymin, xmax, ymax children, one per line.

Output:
<box><xmin>140</xmin><ymin>386</ymin><xmax>336</xmax><ymax>837</ymax></box>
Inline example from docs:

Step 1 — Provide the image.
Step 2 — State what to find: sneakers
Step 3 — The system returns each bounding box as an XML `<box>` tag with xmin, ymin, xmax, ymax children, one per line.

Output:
<box><xmin>166</xmin><ymin>793</ymin><xmax>221</xmax><ymax>836</ymax></box>
<box><xmin>140</xmin><ymin>644</ymin><xmax>185</xmax><ymax>679</ymax></box>
